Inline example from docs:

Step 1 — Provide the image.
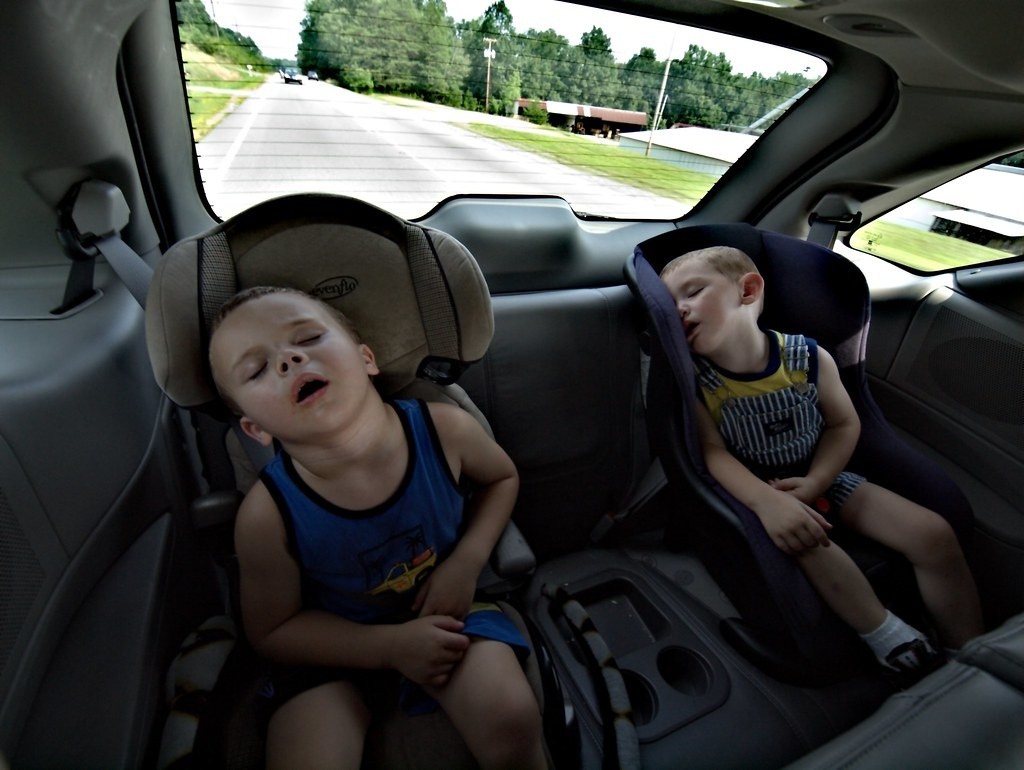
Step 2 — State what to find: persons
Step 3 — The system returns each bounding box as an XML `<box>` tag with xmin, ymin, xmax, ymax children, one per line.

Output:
<box><xmin>206</xmin><ymin>285</ymin><xmax>550</xmax><ymax>770</ymax></box>
<box><xmin>659</xmin><ymin>246</ymin><xmax>984</xmax><ymax>675</ymax></box>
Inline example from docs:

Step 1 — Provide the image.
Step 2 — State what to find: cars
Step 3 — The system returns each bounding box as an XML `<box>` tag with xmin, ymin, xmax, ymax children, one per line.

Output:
<box><xmin>308</xmin><ymin>71</ymin><xmax>319</xmax><ymax>81</ymax></box>
<box><xmin>284</xmin><ymin>66</ymin><xmax>302</xmax><ymax>84</ymax></box>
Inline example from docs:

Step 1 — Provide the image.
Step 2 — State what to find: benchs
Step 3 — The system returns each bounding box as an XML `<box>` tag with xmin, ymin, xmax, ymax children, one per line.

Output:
<box><xmin>790</xmin><ymin>616</ymin><xmax>1024</xmax><ymax>770</ymax></box>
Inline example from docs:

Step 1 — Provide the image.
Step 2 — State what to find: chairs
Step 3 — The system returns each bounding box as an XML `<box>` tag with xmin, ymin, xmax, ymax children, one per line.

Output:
<box><xmin>145</xmin><ymin>192</ymin><xmax>542</xmax><ymax>770</ymax></box>
<box><xmin>623</xmin><ymin>223</ymin><xmax>978</xmax><ymax>632</ymax></box>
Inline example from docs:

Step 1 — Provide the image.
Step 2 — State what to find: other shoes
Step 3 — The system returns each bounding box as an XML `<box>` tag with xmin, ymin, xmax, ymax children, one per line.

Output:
<box><xmin>878</xmin><ymin>639</ymin><xmax>947</xmax><ymax>699</ymax></box>
